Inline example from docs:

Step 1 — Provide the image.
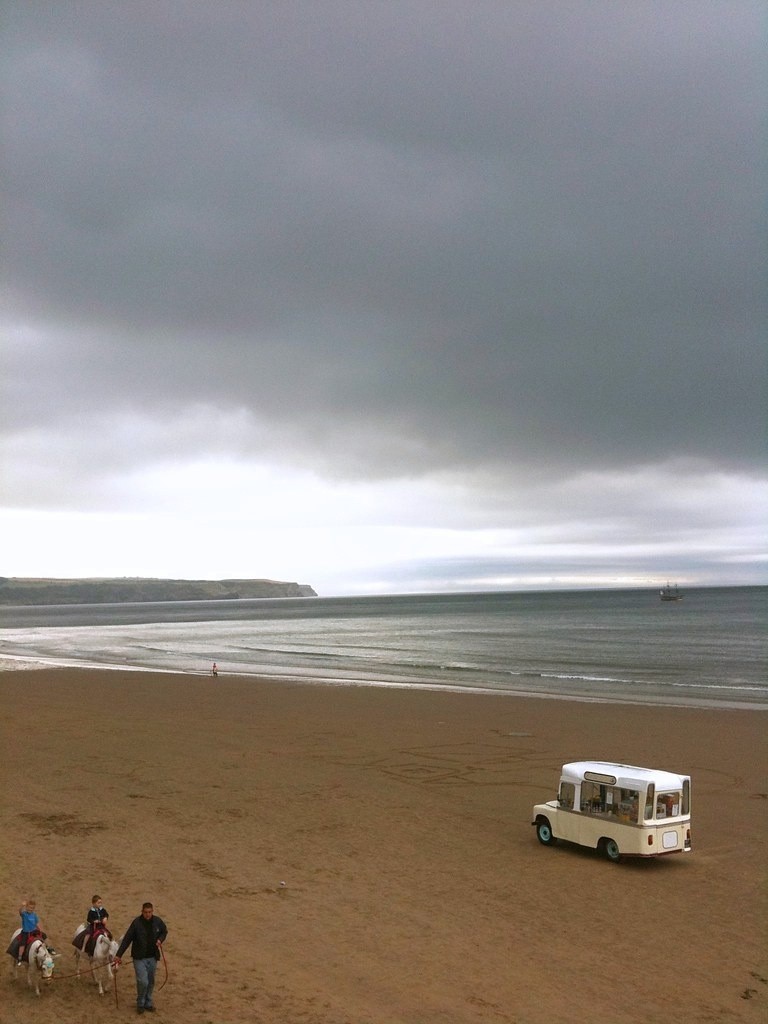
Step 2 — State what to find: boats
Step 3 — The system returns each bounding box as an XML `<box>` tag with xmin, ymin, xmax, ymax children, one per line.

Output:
<box><xmin>658</xmin><ymin>580</ymin><xmax>685</xmax><ymax>601</ymax></box>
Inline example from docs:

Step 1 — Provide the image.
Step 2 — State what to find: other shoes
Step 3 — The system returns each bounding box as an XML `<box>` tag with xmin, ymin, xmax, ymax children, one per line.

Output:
<box><xmin>52</xmin><ymin>951</ymin><xmax>56</xmax><ymax>954</ymax></box>
<box><xmin>145</xmin><ymin>1007</ymin><xmax>156</xmax><ymax>1012</ymax></box>
<box><xmin>17</xmin><ymin>962</ymin><xmax>22</xmax><ymax>966</ymax></box>
<box><xmin>137</xmin><ymin>1007</ymin><xmax>145</xmax><ymax>1015</ymax></box>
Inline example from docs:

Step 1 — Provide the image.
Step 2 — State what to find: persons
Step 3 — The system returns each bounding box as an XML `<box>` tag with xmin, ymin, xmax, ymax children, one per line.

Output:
<box><xmin>18</xmin><ymin>900</ymin><xmax>56</xmax><ymax>966</ymax></box>
<box><xmin>81</xmin><ymin>894</ymin><xmax>114</xmax><ymax>954</ymax></box>
<box><xmin>212</xmin><ymin>663</ymin><xmax>218</xmax><ymax>676</ymax></box>
<box><xmin>112</xmin><ymin>902</ymin><xmax>169</xmax><ymax>1014</ymax></box>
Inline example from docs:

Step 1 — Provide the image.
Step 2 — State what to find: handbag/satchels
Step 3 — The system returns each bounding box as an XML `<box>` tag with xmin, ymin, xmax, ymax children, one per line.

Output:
<box><xmin>154</xmin><ymin>949</ymin><xmax>160</xmax><ymax>961</ymax></box>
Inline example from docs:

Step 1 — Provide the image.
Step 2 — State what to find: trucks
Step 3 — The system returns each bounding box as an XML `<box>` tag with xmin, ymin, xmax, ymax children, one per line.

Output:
<box><xmin>531</xmin><ymin>760</ymin><xmax>693</xmax><ymax>864</ymax></box>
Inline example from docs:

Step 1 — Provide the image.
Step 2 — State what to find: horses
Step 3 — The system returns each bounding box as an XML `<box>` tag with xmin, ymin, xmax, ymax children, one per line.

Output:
<box><xmin>73</xmin><ymin>923</ymin><xmax>126</xmax><ymax>996</ymax></box>
<box><xmin>8</xmin><ymin>928</ymin><xmax>62</xmax><ymax>997</ymax></box>
